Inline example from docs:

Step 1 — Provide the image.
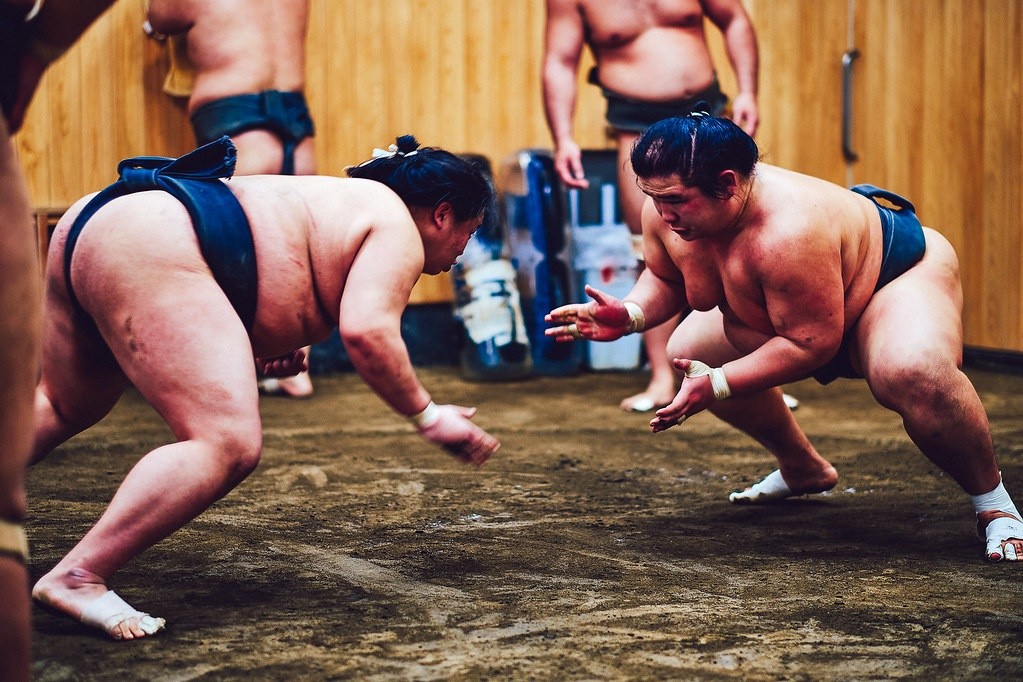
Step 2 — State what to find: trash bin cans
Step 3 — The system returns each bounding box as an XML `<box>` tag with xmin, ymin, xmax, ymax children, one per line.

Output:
<box><xmin>556</xmin><ymin>226</ymin><xmax>641</xmax><ymax>369</ymax></box>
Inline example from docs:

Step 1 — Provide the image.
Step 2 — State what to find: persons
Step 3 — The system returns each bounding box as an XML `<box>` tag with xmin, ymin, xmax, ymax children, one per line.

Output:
<box><xmin>0</xmin><ymin>0</ymin><xmax>108</xmax><ymax>682</ymax></box>
<box><xmin>541</xmin><ymin>0</ymin><xmax>800</xmax><ymax>413</ymax></box>
<box><xmin>148</xmin><ymin>1</ymin><xmax>315</xmax><ymax>396</ymax></box>
<box><xmin>544</xmin><ymin>101</ymin><xmax>1023</xmax><ymax>564</ymax></box>
<box><xmin>23</xmin><ymin>135</ymin><xmax>502</xmax><ymax>641</ymax></box>
